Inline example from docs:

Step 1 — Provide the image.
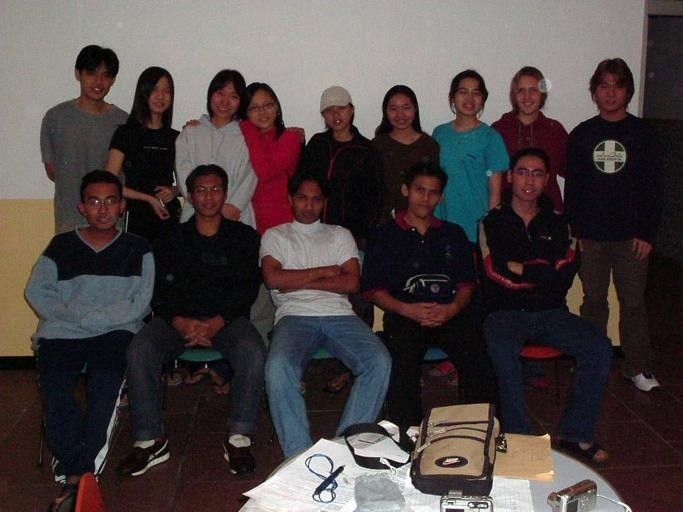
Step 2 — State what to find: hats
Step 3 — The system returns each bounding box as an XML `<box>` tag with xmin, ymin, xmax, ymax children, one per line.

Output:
<box><xmin>320</xmin><ymin>87</ymin><xmax>353</xmax><ymax>113</ymax></box>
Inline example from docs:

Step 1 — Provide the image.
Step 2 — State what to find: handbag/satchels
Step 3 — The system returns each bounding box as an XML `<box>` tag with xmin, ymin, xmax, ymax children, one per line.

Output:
<box><xmin>410</xmin><ymin>402</ymin><xmax>508</xmax><ymax>496</ymax></box>
<box><xmin>398</xmin><ymin>274</ymin><xmax>453</xmax><ymax>304</ymax></box>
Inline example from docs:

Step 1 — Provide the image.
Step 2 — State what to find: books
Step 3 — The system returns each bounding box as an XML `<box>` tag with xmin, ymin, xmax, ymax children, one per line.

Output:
<box><xmin>492</xmin><ymin>432</ymin><xmax>554</xmax><ymax>481</ymax></box>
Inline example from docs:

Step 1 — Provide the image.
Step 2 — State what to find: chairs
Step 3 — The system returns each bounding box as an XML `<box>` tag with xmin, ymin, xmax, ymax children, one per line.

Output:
<box><xmin>523</xmin><ymin>346</ymin><xmax>564</xmax><ymax>405</ymax></box>
<box><xmin>164</xmin><ymin>350</ymin><xmax>232</xmax><ymax>421</ymax></box>
<box><xmin>420</xmin><ymin>348</ymin><xmax>462</xmax><ymax>401</ymax></box>
<box><xmin>304</xmin><ymin>346</ymin><xmax>356</xmax><ymax>393</ymax></box>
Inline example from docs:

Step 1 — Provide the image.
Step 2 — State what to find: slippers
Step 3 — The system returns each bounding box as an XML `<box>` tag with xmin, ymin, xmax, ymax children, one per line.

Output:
<box><xmin>119</xmin><ymin>387</ymin><xmax>128</xmax><ymax>407</ymax></box>
<box><xmin>211</xmin><ymin>380</ymin><xmax>230</xmax><ymax>396</ymax></box>
<box><xmin>51</xmin><ymin>474</ymin><xmax>112</xmax><ymax>512</ymax></box>
<box><xmin>555</xmin><ymin>437</ymin><xmax>609</xmax><ymax>463</ymax></box>
<box><xmin>168</xmin><ymin>369</ymin><xmax>183</xmax><ymax>386</ymax></box>
<box><xmin>183</xmin><ymin>369</ymin><xmax>208</xmax><ymax>385</ymax></box>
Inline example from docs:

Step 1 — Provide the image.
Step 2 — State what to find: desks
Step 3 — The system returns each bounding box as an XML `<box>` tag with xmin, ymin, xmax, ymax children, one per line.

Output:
<box><xmin>236</xmin><ymin>423</ymin><xmax>631</xmax><ymax>512</ymax></box>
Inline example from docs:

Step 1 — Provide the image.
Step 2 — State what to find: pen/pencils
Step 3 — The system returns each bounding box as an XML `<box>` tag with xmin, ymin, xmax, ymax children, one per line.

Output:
<box><xmin>316</xmin><ymin>466</ymin><xmax>345</xmax><ymax>495</ymax></box>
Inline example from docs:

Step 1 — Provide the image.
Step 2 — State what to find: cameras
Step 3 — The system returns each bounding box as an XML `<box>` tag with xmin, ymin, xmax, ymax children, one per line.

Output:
<box><xmin>546</xmin><ymin>479</ymin><xmax>597</xmax><ymax>512</ymax></box>
<box><xmin>440</xmin><ymin>489</ymin><xmax>493</xmax><ymax>512</ymax></box>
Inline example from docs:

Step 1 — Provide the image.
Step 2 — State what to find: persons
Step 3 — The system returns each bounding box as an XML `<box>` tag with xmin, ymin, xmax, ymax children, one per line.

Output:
<box><xmin>104</xmin><ymin>66</ymin><xmax>183</xmax><ymax>408</ymax></box>
<box><xmin>490</xmin><ymin>66</ymin><xmax>569</xmax><ymax>389</ymax></box>
<box><xmin>257</xmin><ymin>170</ymin><xmax>393</xmax><ymax>459</ymax></box>
<box><xmin>115</xmin><ymin>165</ymin><xmax>267</xmax><ymax>479</ymax></box>
<box><xmin>359</xmin><ymin>161</ymin><xmax>497</xmax><ymax>433</ymax></box>
<box><xmin>286</xmin><ymin>86</ymin><xmax>390</xmax><ymax>395</ymax></box>
<box><xmin>39</xmin><ymin>44</ymin><xmax>132</xmax><ymax>235</ymax></box>
<box><xmin>173</xmin><ymin>70</ymin><xmax>259</xmax><ymax>394</ymax></box>
<box><xmin>562</xmin><ymin>58</ymin><xmax>662</xmax><ymax>392</ymax></box>
<box><xmin>475</xmin><ymin>147</ymin><xmax>614</xmax><ymax>463</ymax></box>
<box><xmin>427</xmin><ymin>69</ymin><xmax>510</xmax><ymax>388</ymax></box>
<box><xmin>370</xmin><ymin>85</ymin><xmax>440</xmax><ymax>393</ymax></box>
<box><xmin>24</xmin><ymin>169</ymin><xmax>155</xmax><ymax>512</ymax></box>
<box><xmin>182</xmin><ymin>82</ymin><xmax>308</xmax><ymax>401</ymax></box>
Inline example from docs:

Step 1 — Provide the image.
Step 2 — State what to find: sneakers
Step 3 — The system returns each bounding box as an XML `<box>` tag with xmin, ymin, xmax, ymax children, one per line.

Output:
<box><xmin>528</xmin><ymin>376</ymin><xmax>558</xmax><ymax>395</ymax></box>
<box><xmin>222</xmin><ymin>431</ymin><xmax>257</xmax><ymax>476</ymax></box>
<box><xmin>623</xmin><ymin>370</ymin><xmax>660</xmax><ymax>392</ymax></box>
<box><xmin>428</xmin><ymin>361</ymin><xmax>456</xmax><ymax>378</ymax></box>
<box><xmin>116</xmin><ymin>435</ymin><xmax>171</xmax><ymax>476</ymax></box>
<box><xmin>324</xmin><ymin>372</ymin><xmax>351</xmax><ymax>400</ymax></box>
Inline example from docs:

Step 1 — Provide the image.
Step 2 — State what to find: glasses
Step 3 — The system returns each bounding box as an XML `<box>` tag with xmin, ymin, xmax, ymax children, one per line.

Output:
<box><xmin>194</xmin><ymin>184</ymin><xmax>223</xmax><ymax>195</ymax></box>
<box><xmin>514</xmin><ymin>87</ymin><xmax>542</xmax><ymax>98</ymax></box>
<box><xmin>246</xmin><ymin>100</ymin><xmax>276</xmax><ymax>112</ymax></box>
<box><xmin>515</xmin><ymin>169</ymin><xmax>545</xmax><ymax>181</ymax></box>
<box><xmin>85</xmin><ymin>197</ymin><xmax>121</xmax><ymax>209</ymax></box>
